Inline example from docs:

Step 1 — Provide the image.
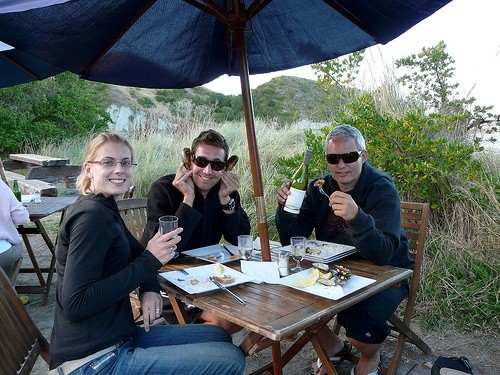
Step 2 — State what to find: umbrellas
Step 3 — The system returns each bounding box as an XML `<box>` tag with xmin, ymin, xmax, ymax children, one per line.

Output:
<box><xmin>0</xmin><ymin>0</ymin><xmax>452</xmax><ymax>262</ymax></box>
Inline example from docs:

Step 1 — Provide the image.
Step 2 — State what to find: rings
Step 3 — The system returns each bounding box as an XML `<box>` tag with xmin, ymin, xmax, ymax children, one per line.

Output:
<box><xmin>171</xmin><ymin>248</ymin><xmax>172</xmax><ymax>253</ymax></box>
<box><xmin>156</xmin><ymin>309</ymin><xmax>161</xmax><ymax>314</ymax></box>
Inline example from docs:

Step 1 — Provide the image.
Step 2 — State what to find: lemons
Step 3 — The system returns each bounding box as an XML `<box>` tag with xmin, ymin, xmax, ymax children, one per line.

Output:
<box><xmin>320</xmin><ymin>272</ymin><xmax>332</xmax><ymax>279</ymax></box>
<box><xmin>216</xmin><ymin>264</ymin><xmax>225</xmax><ymax>275</ymax></box>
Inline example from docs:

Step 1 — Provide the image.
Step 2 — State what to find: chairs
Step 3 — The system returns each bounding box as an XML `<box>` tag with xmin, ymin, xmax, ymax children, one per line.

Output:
<box><xmin>117</xmin><ymin>198</ymin><xmax>148</xmax><ymax>320</ymax></box>
<box><xmin>0</xmin><ymin>257</ymin><xmax>50</xmax><ymax>375</ymax></box>
<box><xmin>333</xmin><ymin>202</ymin><xmax>433</xmax><ymax>375</ymax></box>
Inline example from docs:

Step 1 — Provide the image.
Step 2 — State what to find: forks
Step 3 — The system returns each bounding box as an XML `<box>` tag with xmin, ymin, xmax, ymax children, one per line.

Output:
<box><xmin>318</xmin><ymin>184</ymin><xmax>330</xmax><ymax>199</ymax></box>
<box><xmin>158</xmin><ymin>269</ymin><xmax>189</xmax><ymax>275</ymax></box>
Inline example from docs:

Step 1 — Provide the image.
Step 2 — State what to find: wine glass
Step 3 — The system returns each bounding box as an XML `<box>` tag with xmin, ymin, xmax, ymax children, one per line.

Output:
<box><xmin>159</xmin><ymin>216</ymin><xmax>180</xmax><ymax>259</ymax></box>
<box><xmin>290</xmin><ymin>237</ymin><xmax>307</xmax><ymax>272</ymax></box>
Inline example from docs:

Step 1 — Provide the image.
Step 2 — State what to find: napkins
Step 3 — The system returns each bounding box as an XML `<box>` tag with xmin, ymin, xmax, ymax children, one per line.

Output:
<box><xmin>21</xmin><ymin>195</ymin><xmax>41</xmax><ymax>203</ymax></box>
<box><xmin>240</xmin><ymin>260</ymin><xmax>280</xmax><ymax>284</ymax></box>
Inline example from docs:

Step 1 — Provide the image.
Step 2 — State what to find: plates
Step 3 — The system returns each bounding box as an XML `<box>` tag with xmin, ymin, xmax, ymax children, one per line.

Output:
<box><xmin>158</xmin><ymin>263</ymin><xmax>255</xmax><ymax>294</ymax></box>
<box><xmin>181</xmin><ymin>243</ymin><xmax>241</xmax><ymax>264</ymax></box>
<box><xmin>276</xmin><ymin>267</ymin><xmax>376</xmax><ymax>300</ymax></box>
<box><xmin>278</xmin><ymin>240</ymin><xmax>357</xmax><ymax>263</ymax></box>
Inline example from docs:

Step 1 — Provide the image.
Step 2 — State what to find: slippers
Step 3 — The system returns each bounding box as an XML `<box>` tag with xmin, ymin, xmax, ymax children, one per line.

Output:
<box><xmin>350</xmin><ymin>354</ymin><xmax>383</xmax><ymax>375</ymax></box>
<box><xmin>310</xmin><ymin>340</ymin><xmax>353</xmax><ymax>375</ymax></box>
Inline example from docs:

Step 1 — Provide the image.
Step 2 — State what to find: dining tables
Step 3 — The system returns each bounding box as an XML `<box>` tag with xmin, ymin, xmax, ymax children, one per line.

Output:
<box><xmin>161</xmin><ymin>246</ymin><xmax>414</xmax><ymax>375</ymax></box>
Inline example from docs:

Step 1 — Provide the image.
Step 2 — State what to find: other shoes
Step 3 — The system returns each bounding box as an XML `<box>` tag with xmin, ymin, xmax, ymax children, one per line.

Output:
<box><xmin>20</xmin><ymin>295</ymin><xmax>29</xmax><ymax>306</ymax></box>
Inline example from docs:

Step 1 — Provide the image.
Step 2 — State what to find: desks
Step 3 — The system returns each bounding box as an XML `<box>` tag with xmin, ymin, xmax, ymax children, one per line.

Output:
<box><xmin>16</xmin><ymin>197</ymin><xmax>78</xmax><ymax>305</ymax></box>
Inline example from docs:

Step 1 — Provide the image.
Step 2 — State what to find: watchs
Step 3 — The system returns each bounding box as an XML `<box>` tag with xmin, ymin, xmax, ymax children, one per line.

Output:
<box><xmin>222</xmin><ymin>199</ymin><xmax>235</xmax><ymax>210</ymax></box>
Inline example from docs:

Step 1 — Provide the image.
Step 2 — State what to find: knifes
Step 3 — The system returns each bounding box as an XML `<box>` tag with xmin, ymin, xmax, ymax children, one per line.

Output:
<box><xmin>209</xmin><ymin>277</ymin><xmax>246</xmax><ymax>306</ymax></box>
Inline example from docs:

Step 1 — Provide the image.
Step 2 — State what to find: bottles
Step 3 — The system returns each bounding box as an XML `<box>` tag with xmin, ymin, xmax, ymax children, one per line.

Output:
<box><xmin>13</xmin><ymin>179</ymin><xmax>21</xmax><ymax>203</ymax></box>
<box><xmin>280</xmin><ymin>149</ymin><xmax>313</xmax><ymax>218</ymax></box>
<box><xmin>277</xmin><ymin>250</ymin><xmax>290</xmax><ymax>277</ymax></box>
<box><xmin>35</xmin><ymin>191</ymin><xmax>41</xmax><ymax>203</ymax></box>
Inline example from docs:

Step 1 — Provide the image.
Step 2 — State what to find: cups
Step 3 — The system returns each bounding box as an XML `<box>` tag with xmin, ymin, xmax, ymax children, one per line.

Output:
<box><xmin>238</xmin><ymin>235</ymin><xmax>253</xmax><ymax>260</ymax></box>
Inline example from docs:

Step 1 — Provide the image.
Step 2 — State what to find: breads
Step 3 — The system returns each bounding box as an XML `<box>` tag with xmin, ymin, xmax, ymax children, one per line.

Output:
<box><xmin>212</xmin><ymin>276</ymin><xmax>235</xmax><ymax>284</ymax></box>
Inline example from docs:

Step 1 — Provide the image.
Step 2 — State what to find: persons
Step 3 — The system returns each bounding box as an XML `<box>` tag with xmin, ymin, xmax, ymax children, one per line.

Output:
<box><xmin>275</xmin><ymin>125</ymin><xmax>415</xmax><ymax>375</ymax></box>
<box><xmin>0</xmin><ymin>177</ymin><xmax>29</xmax><ymax>267</ymax></box>
<box><xmin>141</xmin><ymin>130</ymin><xmax>251</xmax><ymax>333</ymax></box>
<box><xmin>48</xmin><ymin>132</ymin><xmax>245</xmax><ymax>375</ymax></box>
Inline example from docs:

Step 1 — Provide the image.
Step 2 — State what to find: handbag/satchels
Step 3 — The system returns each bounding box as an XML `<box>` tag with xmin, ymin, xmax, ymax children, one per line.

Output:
<box><xmin>431</xmin><ymin>355</ymin><xmax>481</xmax><ymax>375</ymax></box>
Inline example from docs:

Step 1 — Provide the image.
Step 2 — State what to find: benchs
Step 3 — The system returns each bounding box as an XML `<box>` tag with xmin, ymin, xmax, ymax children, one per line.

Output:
<box><xmin>3</xmin><ymin>154</ymin><xmax>82</xmax><ymax>180</ymax></box>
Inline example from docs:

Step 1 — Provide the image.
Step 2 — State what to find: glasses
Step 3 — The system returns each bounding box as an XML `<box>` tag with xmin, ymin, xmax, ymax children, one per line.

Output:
<box><xmin>325</xmin><ymin>150</ymin><xmax>363</xmax><ymax>165</ymax></box>
<box><xmin>191</xmin><ymin>155</ymin><xmax>227</xmax><ymax>171</ymax></box>
<box><xmin>87</xmin><ymin>158</ymin><xmax>138</xmax><ymax>170</ymax></box>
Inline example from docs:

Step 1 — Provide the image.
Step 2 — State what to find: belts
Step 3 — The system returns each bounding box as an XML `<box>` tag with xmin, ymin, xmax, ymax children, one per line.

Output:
<box><xmin>48</xmin><ymin>342</ymin><xmax>120</xmax><ymax>375</ymax></box>
